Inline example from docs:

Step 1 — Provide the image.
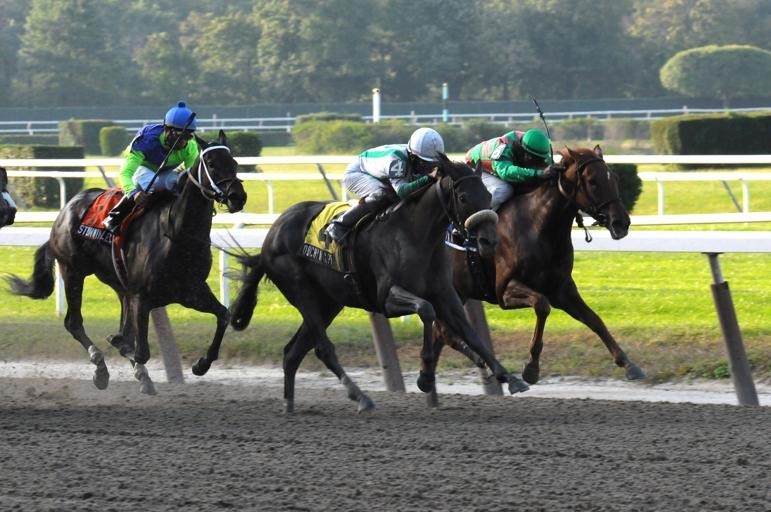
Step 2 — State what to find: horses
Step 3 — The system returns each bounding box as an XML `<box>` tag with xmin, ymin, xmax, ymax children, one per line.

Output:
<box><xmin>424</xmin><ymin>144</ymin><xmax>645</xmax><ymax>408</ymax></box>
<box><xmin>1</xmin><ymin>128</ymin><xmax>248</xmax><ymax>396</ymax></box>
<box><xmin>213</xmin><ymin>149</ymin><xmax>530</xmax><ymax>416</ymax></box>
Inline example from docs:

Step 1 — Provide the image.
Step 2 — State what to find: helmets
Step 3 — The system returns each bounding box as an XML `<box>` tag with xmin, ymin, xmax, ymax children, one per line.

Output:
<box><xmin>519</xmin><ymin>127</ymin><xmax>550</xmax><ymax>160</ymax></box>
<box><xmin>162</xmin><ymin>98</ymin><xmax>198</xmax><ymax>131</ymax></box>
<box><xmin>405</xmin><ymin>126</ymin><xmax>445</xmax><ymax>164</ymax></box>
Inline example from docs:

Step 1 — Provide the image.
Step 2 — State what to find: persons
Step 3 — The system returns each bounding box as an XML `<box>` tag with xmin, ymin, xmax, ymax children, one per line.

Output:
<box><xmin>102</xmin><ymin>101</ymin><xmax>200</xmax><ymax>234</ymax></box>
<box><xmin>465</xmin><ymin>129</ymin><xmax>557</xmax><ymax>211</ymax></box>
<box><xmin>325</xmin><ymin>127</ymin><xmax>444</xmax><ymax>247</ymax></box>
